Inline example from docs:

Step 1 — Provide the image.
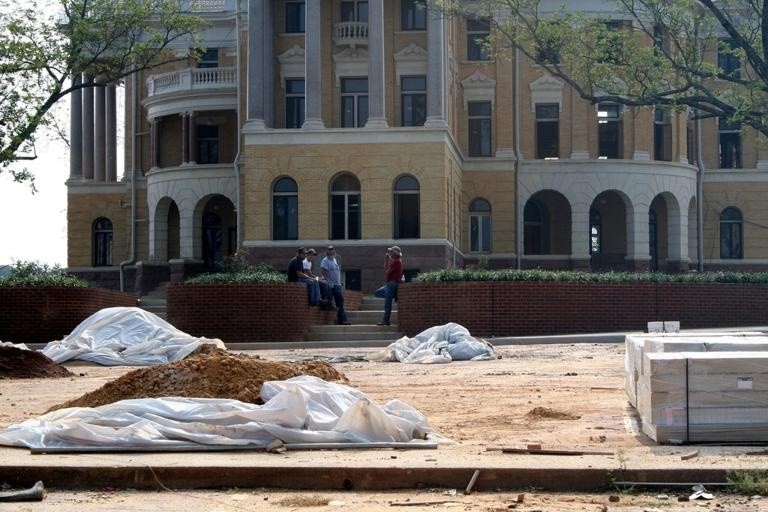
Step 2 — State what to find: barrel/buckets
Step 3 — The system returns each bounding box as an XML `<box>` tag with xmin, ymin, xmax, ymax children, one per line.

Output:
<box><xmin>664</xmin><ymin>320</ymin><xmax>680</xmax><ymax>333</ymax></box>
<box><xmin>647</xmin><ymin>321</ymin><xmax>664</xmax><ymax>333</ymax></box>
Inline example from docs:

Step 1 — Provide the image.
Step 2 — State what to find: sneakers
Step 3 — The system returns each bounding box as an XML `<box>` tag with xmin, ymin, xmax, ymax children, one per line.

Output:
<box><xmin>394</xmin><ymin>291</ymin><xmax>398</xmax><ymax>302</ymax></box>
<box><xmin>377</xmin><ymin>319</ymin><xmax>390</xmax><ymax>325</ymax></box>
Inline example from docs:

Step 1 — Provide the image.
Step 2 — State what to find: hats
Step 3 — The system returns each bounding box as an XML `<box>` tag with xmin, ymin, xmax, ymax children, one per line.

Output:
<box><xmin>307</xmin><ymin>248</ymin><xmax>317</xmax><ymax>256</ymax></box>
<box><xmin>388</xmin><ymin>246</ymin><xmax>403</xmax><ymax>257</ymax></box>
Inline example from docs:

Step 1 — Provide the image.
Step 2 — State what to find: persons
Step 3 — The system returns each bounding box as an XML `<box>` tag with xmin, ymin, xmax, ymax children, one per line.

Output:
<box><xmin>303</xmin><ymin>248</ymin><xmax>339</xmax><ymax>311</ymax></box>
<box><xmin>320</xmin><ymin>245</ymin><xmax>352</xmax><ymax>325</ymax></box>
<box><xmin>286</xmin><ymin>246</ymin><xmax>326</xmax><ymax>307</ymax></box>
<box><xmin>375</xmin><ymin>246</ymin><xmax>405</xmax><ymax>326</ymax></box>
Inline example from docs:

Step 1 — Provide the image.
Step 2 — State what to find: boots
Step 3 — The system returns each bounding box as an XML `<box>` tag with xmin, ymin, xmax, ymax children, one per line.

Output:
<box><xmin>0</xmin><ymin>480</ymin><xmax>44</xmax><ymax>502</ymax></box>
